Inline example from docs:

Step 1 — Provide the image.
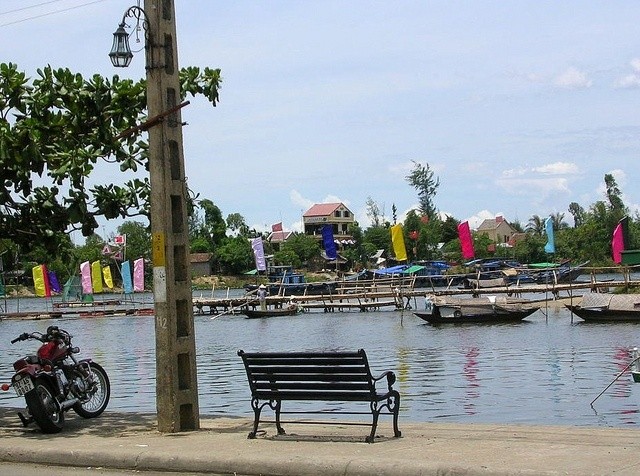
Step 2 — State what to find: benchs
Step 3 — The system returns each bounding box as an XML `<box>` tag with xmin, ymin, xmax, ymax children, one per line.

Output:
<box><xmin>237</xmin><ymin>349</ymin><xmax>402</xmax><ymax>443</ymax></box>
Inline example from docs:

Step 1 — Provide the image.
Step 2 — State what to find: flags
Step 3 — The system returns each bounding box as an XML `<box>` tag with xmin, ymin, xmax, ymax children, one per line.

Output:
<box><xmin>48</xmin><ymin>269</ymin><xmax>61</xmax><ymax>292</ymax></box>
<box><xmin>120</xmin><ymin>259</ymin><xmax>134</xmax><ymax>293</ymax></box>
<box><xmin>251</xmin><ymin>236</ymin><xmax>266</xmax><ymax>271</ymax></box>
<box><xmin>320</xmin><ymin>224</ymin><xmax>337</xmax><ymax>259</ymax></box>
<box><xmin>612</xmin><ymin>222</ymin><xmax>625</xmax><ymax>264</ymax></box>
<box><xmin>41</xmin><ymin>263</ymin><xmax>52</xmax><ymax>296</ymax></box>
<box><xmin>272</xmin><ymin>221</ymin><xmax>282</xmax><ymax>231</ymax></box>
<box><xmin>113</xmin><ymin>236</ymin><xmax>125</xmax><ymax>244</ymax></box>
<box><xmin>102</xmin><ymin>265</ymin><xmax>114</xmax><ymax>289</ymax></box>
<box><xmin>457</xmin><ymin>220</ymin><xmax>475</xmax><ymax>258</ymax></box>
<box><xmin>391</xmin><ymin>222</ymin><xmax>408</xmax><ymax>261</ymax></box>
<box><xmin>133</xmin><ymin>257</ymin><xmax>145</xmax><ymax>292</ymax></box>
<box><xmin>91</xmin><ymin>259</ymin><xmax>103</xmax><ymax>293</ymax></box>
<box><xmin>80</xmin><ymin>260</ymin><xmax>93</xmax><ymax>293</ymax></box>
<box><xmin>544</xmin><ymin>215</ymin><xmax>555</xmax><ymax>253</ymax></box>
<box><xmin>31</xmin><ymin>263</ymin><xmax>46</xmax><ymax>297</ymax></box>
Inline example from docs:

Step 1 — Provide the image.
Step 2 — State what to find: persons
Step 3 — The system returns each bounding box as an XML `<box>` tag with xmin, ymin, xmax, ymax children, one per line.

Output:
<box><xmin>257</xmin><ymin>282</ymin><xmax>267</xmax><ymax>310</ymax></box>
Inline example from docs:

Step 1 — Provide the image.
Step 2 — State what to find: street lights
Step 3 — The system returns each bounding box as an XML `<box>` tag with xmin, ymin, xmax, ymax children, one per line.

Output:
<box><xmin>110</xmin><ymin>7</ymin><xmax>199</xmax><ymax>433</ymax></box>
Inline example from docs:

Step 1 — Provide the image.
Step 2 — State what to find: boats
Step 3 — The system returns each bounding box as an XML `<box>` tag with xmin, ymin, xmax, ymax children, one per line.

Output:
<box><xmin>415</xmin><ymin>305</ymin><xmax>540</xmax><ymax>324</ymax></box>
<box><xmin>458</xmin><ymin>279</ymin><xmax>514</xmax><ymax>289</ymax></box>
<box><xmin>242</xmin><ymin>306</ymin><xmax>300</xmax><ymax>319</ymax></box>
<box><xmin>532</xmin><ymin>261</ymin><xmax>591</xmax><ymax>283</ymax></box>
<box><xmin>564</xmin><ymin>294</ymin><xmax>640</xmax><ymax>322</ymax></box>
<box><xmin>243</xmin><ymin>266</ymin><xmax>346</xmax><ymax>295</ymax></box>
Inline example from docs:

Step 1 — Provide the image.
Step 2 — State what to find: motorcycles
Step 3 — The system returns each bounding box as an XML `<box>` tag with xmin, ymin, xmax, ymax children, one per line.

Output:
<box><xmin>2</xmin><ymin>326</ymin><xmax>110</xmax><ymax>432</ymax></box>
